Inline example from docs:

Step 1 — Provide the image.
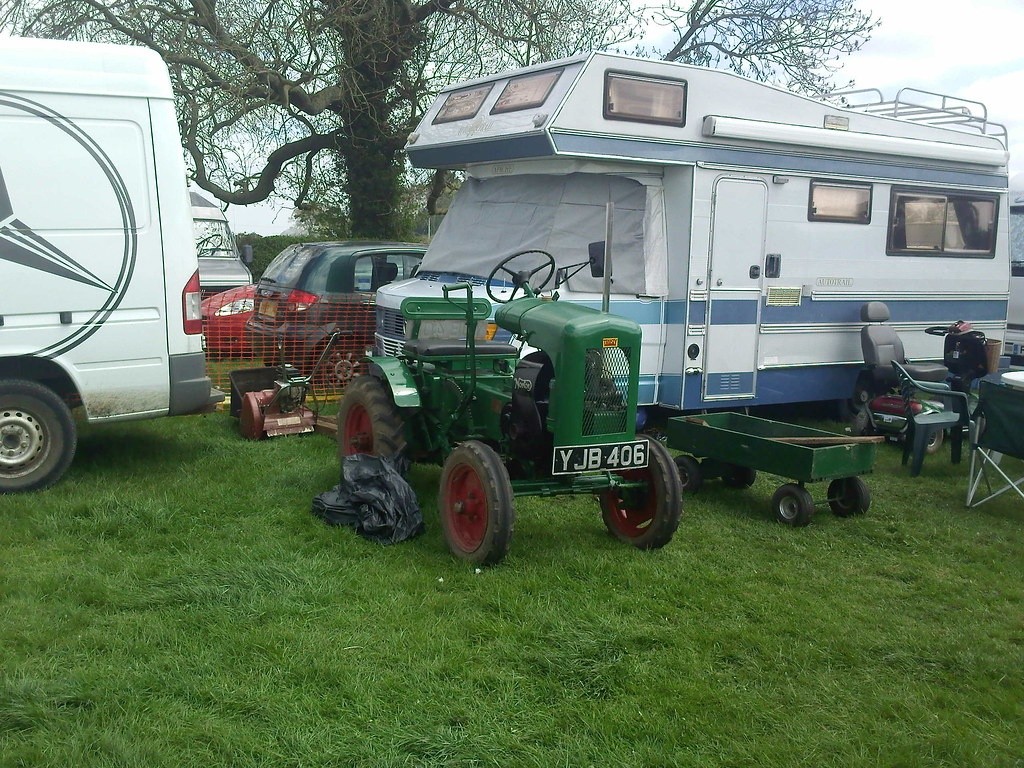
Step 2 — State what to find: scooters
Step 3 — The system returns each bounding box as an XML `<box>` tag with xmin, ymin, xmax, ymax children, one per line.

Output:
<box><xmin>849</xmin><ymin>301</ymin><xmax>1003</xmax><ymax>455</ymax></box>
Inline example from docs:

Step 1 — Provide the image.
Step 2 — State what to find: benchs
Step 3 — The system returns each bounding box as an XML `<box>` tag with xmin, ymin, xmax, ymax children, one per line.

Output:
<box><xmin>402</xmin><ymin>296</ymin><xmax>517</xmax><ymax>359</ymax></box>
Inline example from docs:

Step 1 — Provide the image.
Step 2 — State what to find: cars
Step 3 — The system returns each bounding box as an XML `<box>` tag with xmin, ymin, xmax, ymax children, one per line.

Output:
<box><xmin>197</xmin><ymin>282</ymin><xmax>258</xmax><ymax>360</ymax></box>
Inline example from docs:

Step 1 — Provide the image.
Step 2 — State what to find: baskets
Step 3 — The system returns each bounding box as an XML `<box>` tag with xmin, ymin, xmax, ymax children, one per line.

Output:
<box><xmin>984</xmin><ymin>337</ymin><xmax>1002</xmax><ymax>374</ymax></box>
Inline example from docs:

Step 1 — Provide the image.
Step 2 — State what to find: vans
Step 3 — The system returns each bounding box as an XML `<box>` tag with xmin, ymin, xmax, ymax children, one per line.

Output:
<box><xmin>1</xmin><ymin>36</ymin><xmax>226</xmax><ymax>500</ymax></box>
<box><xmin>188</xmin><ymin>188</ymin><xmax>255</xmax><ymax>300</ymax></box>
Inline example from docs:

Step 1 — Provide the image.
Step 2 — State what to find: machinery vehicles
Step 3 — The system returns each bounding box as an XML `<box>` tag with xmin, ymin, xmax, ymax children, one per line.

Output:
<box><xmin>337</xmin><ymin>246</ymin><xmax>686</xmax><ymax>571</ymax></box>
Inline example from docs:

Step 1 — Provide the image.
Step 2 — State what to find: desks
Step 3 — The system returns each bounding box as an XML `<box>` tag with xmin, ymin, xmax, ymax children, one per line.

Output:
<box><xmin>964</xmin><ymin>369</ymin><xmax>1024</xmax><ymax>508</ymax></box>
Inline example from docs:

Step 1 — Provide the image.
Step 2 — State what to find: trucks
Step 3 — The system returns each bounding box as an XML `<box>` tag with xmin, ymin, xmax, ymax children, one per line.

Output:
<box><xmin>359</xmin><ymin>49</ymin><xmax>1011</xmax><ymax>434</ymax></box>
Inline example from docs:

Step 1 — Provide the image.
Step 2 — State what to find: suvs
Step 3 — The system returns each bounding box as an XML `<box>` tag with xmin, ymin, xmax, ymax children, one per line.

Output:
<box><xmin>246</xmin><ymin>239</ymin><xmax>432</xmax><ymax>392</ymax></box>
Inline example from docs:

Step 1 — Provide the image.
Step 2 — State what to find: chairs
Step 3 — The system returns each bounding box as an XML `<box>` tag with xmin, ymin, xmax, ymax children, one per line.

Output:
<box><xmin>889</xmin><ymin>360</ymin><xmax>970</xmax><ymax>475</ymax></box>
<box><xmin>378</xmin><ymin>260</ymin><xmax>397</xmax><ymax>288</ymax></box>
